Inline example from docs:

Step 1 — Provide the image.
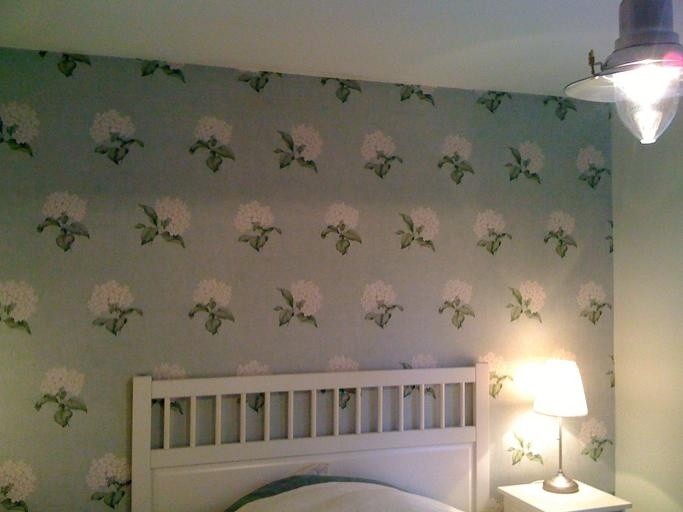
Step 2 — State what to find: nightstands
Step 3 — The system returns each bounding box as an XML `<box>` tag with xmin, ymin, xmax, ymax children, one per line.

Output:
<box><xmin>499</xmin><ymin>478</ymin><xmax>633</xmax><ymax>512</ymax></box>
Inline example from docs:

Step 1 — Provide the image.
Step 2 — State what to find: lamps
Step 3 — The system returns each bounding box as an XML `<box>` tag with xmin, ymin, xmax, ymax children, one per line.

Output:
<box><xmin>562</xmin><ymin>2</ymin><xmax>683</xmax><ymax>147</ymax></box>
<box><xmin>535</xmin><ymin>357</ymin><xmax>589</xmax><ymax>494</ymax></box>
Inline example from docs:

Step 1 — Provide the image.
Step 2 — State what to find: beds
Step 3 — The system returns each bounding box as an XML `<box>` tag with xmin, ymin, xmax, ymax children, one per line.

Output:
<box><xmin>123</xmin><ymin>358</ymin><xmax>490</xmax><ymax>512</ymax></box>
